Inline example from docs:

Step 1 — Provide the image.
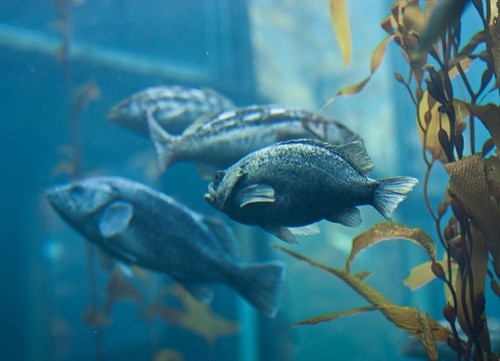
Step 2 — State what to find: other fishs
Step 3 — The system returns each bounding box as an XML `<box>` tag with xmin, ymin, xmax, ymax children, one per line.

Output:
<box><xmin>45</xmin><ymin>176</ymin><xmax>286</xmax><ymax>322</ymax></box>
<box><xmin>204</xmin><ymin>137</ymin><xmax>419</xmax><ymax>245</ymax></box>
<box><xmin>108</xmin><ymin>85</ymin><xmax>368</xmax><ymax>175</ymax></box>
